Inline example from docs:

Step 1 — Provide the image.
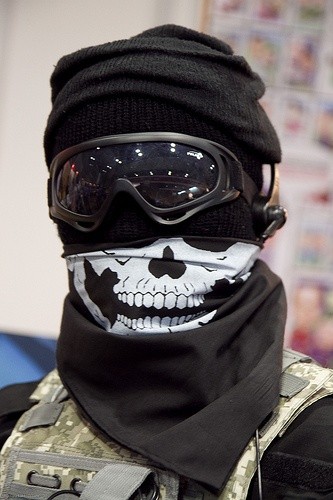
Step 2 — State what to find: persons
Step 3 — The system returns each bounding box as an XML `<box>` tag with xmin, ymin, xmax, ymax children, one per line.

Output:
<box><xmin>0</xmin><ymin>22</ymin><xmax>333</xmax><ymax>499</ymax></box>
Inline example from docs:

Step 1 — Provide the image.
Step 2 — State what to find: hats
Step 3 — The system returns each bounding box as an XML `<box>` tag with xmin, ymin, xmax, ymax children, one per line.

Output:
<box><xmin>41</xmin><ymin>25</ymin><xmax>284</xmax><ymax>179</ymax></box>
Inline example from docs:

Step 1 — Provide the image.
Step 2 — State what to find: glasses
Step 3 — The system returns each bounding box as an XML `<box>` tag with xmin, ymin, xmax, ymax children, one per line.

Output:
<box><xmin>44</xmin><ymin>130</ymin><xmax>262</xmax><ymax>232</ymax></box>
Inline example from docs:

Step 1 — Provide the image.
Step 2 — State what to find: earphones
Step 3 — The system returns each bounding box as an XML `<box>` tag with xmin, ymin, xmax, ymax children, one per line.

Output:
<box><xmin>252</xmin><ymin>195</ymin><xmax>288</xmax><ymax>244</ymax></box>
